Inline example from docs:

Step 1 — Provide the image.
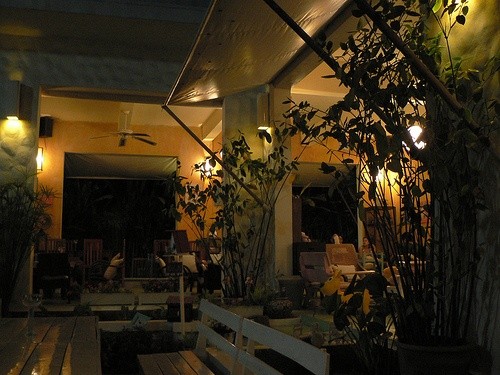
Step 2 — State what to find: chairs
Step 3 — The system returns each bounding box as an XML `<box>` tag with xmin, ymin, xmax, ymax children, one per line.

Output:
<box><xmin>326</xmin><ymin>243</ymin><xmax>376</xmax><ymax>286</ymax></box>
<box><xmin>299</xmin><ymin>251</ymin><xmax>357</xmax><ymax>310</ymax></box>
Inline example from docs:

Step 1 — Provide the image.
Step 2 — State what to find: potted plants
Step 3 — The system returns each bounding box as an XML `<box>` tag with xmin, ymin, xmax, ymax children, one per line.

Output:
<box><xmin>280</xmin><ymin>0</ymin><xmax>500</xmax><ymax>375</ymax></box>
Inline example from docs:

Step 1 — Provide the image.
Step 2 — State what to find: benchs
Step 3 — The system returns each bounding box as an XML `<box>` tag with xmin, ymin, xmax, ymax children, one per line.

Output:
<box><xmin>136</xmin><ymin>298</ymin><xmax>330</xmax><ymax>375</ymax></box>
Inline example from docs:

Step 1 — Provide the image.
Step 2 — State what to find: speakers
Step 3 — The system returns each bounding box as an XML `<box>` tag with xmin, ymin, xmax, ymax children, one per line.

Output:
<box><xmin>39</xmin><ymin>116</ymin><xmax>52</xmax><ymax>138</ymax></box>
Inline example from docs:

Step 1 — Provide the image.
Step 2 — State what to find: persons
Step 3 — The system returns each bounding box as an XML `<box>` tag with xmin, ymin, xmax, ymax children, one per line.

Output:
<box><xmin>296</xmin><ymin>232</ymin><xmax>345</xmax><ymax>252</ymax></box>
<box><xmin>359</xmin><ymin>237</ymin><xmax>378</xmax><ymax>269</ymax></box>
<box><xmin>391</xmin><ymin>232</ymin><xmax>431</xmax><ymax>261</ymax></box>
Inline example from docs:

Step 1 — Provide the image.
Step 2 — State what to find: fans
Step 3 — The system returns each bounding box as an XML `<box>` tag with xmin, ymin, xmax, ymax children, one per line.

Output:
<box><xmin>89</xmin><ymin>109</ymin><xmax>157</xmax><ymax>147</ymax></box>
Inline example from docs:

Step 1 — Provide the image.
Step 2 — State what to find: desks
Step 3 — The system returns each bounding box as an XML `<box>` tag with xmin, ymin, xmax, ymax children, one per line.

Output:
<box><xmin>1</xmin><ymin>317</ymin><xmax>100</xmax><ymax>375</ymax></box>
